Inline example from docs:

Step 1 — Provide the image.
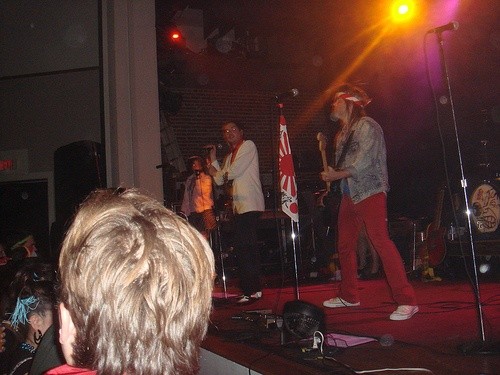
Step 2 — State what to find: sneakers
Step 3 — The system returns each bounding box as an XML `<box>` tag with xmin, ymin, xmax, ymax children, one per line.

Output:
<box><xmin>323</xmin><ymin>296</ymin><xmax>363</xmax><ymax>308</ymax></box>
<box><xmin>389</xmin><ymin>303</ymin><xmax>419</xmax><ymax>321</ymax></box>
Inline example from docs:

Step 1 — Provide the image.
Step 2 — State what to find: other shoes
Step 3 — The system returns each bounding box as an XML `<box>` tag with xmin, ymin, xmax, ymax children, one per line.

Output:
<box><xmin>234</xmin><ymin>288</ymin><xmax>263</xmax><ymax>307</ymax></box>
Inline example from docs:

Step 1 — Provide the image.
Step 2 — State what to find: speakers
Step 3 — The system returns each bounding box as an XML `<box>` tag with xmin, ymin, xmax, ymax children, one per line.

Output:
<box><xmin>53</xmin><ymin>141</ymin><xmax>106</xmax><ymax>235</ymax></box>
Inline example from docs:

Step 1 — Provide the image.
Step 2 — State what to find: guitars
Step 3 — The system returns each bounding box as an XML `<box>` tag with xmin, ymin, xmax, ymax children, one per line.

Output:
<box><xmin>308</xmin><ymin>132</ymin><xmax>333</xmax><ymax>212</ymax></box>
<box><xmin>419</xmin><ymin>182</ymin><xmax>447</xmax><ymax>267</ymax></box>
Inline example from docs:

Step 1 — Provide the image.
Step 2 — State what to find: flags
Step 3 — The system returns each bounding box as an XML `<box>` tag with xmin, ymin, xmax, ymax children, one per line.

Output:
<box><xmin>277</xmin><ymin>104</ymin><xmax>298</xmax><ymax>222</ymax></box>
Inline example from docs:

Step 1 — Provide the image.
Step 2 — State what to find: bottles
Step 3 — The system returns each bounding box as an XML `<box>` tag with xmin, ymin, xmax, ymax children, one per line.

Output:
<box><xmin>451</xmin><ymin>222</ymin><xmax>455</xmax><ymax>240</ymax></box>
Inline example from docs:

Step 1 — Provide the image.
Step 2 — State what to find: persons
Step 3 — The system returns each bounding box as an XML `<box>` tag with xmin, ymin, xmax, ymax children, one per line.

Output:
<box><xmin>0</xmin><ymin>228</ymin><xmax>66</xmax><ymax>375</ymax></box>
<box><xmin>204</xmin><ymin>119</ymin><xmax>266</xmax><ymax>306</ymax></box>
<box><xmin>42</xmin><ymin>186</ymin><xmax>215</xmax><ymax>375</ymax></box>
<box><xmin>180</xmin><ymin>156</ymin><xmax>215</xmax><ymax>241</ymax></box>
<box><xmin>357</xmin><ymin>221</ymin><xmax>381</xmax><ymax>279</ymax></box>
<box><xmin>320</xmin><ymin>83</ymin><xmax>419</xmax><ymax>321</ymax></box>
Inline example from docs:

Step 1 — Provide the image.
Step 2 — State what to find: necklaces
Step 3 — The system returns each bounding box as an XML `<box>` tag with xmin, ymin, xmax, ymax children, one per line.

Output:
<box><xmin>17</xmin><ymin>342</ymin><xmax>36</xmax><ymax>355</ymax></box>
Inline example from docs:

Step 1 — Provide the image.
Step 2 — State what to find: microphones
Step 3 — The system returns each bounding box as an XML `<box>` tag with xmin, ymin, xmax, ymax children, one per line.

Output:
<box><xmin>275</xmin><ymin>88</ymin><xmax>298</xmax><ymax>101</ymax></box>
<box><xmin>200</xmin><ymin>144</ymin><xmax>222</xmax><ymax>153</ymax></box>
<box><xmin>156</xmin><ymin>162</ymin><xmax>172</xmax><ymax>169</ymax></box>
<box><xmin>428</xmin><ymin>21</ymin><xmax>459</xmax><ymax>34</ymax></box>
